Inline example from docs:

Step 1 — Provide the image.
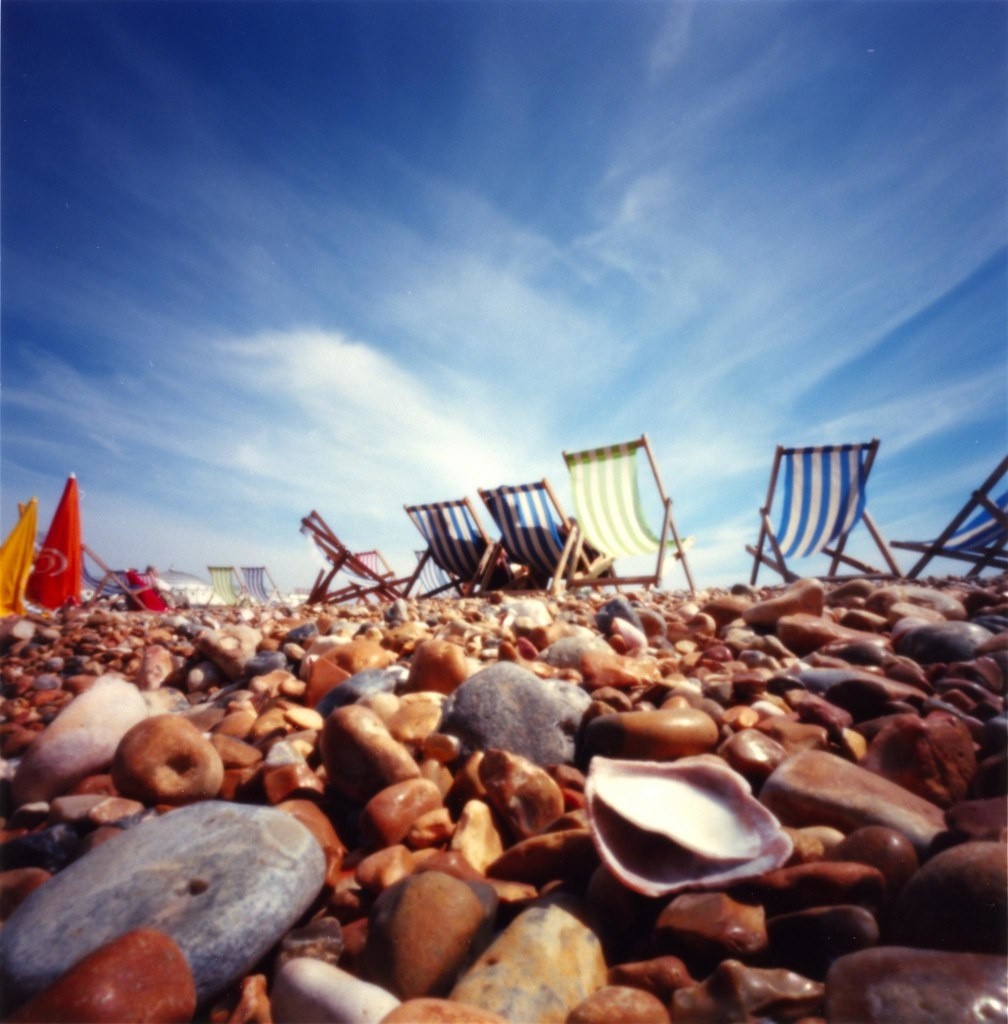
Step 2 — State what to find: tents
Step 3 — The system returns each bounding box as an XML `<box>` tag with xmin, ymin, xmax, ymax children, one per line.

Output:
<box><xmin>155</xmin><ymin>569</ymin><xmax>228</xmax><ymax>606</ymax></box>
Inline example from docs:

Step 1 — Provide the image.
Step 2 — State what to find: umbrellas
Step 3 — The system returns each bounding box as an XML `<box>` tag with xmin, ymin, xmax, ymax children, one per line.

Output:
<box><xmin>25</xmin><ymin>472</ymin><xmax>85</xmax><ymax>607</ymax></box>
<box><xmin>0</xmin><ymin>496</ymin><xmax>39</xmax><ymax>618</ymax></box>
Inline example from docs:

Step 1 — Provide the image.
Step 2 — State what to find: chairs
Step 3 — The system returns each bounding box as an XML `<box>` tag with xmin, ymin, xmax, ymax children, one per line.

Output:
<box><xmin>80</xmin><ymin>542</ymin><xmax>184</xmax><ymax>609</ymax></box>
<box><xmin>299</xmin><ymin>434</ymin><xmax>698</xmax><ymax>607</ymax></box>
<box><xmin>204</xmin><ymin>563</ymin><xmax>287</xmax><ymax>610</ymax></box>
<box><xmin>747</xmin><ymin>437</ymin><xmax>1008</xmax><ymax>587</ymax></box>
